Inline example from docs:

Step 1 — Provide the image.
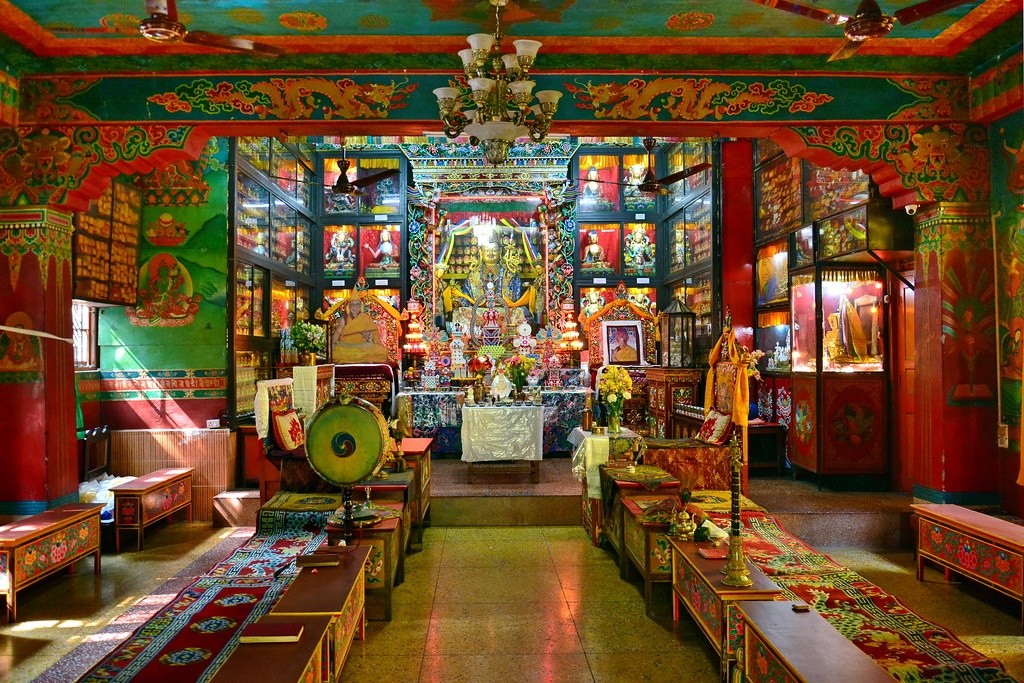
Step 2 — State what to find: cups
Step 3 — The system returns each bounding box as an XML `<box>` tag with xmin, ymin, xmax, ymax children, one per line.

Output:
<box><xmin>591</xmin><ymin>427</ymin><xmax>605</xmax><ymax>436</ymax></box>
<box><xmin>206</xmin><ymin>419</ymin><xmax>220</xmax><ymax>428</ymax></box>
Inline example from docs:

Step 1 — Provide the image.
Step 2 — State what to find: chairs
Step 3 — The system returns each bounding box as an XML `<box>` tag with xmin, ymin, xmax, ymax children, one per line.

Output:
<box><xmin>644</xmin><ymin>304</ymin><xmax>784</xmax><ymax>499</ymax></box>
<box><xmin>254</xmin><ymin>378</ymin><xmax>341</xmax><ymax>494</ymax></box>
<box><xmin>83</xmin><ymin>425</ymin><xmax>114</xmax><ymax>553</ymax></box>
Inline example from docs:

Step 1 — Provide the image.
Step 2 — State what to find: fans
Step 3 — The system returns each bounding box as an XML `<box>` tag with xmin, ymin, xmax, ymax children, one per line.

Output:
<box><xmin>46</xmin><ymin>0</ymin><xmax>285</xmax><ymax>57</ymax></box>
<box><xmin>269</xmin><ymin>143</ymin><xmax>400</xmax><ymax>197</ymax></box>
<box><xmin>576</xmin><ymin>136</ymin><xmax>712</xmax><ymax>195</ymax></box>
<box><xmin>754</xmin><ymin>0</ymin><xmax>975</xmax><ymax>63</ymax></box>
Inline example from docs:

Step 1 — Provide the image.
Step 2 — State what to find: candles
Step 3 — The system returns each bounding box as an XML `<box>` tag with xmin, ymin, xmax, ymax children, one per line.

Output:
<box><xmin>560</xmin><ymin>315</ymin><xmax>583</xmax><ymax>350</ymax></box>
<box><xmin>404</xmin><ymin>315</ymin><xmax>424</xmax><ymax>352</ymax></box>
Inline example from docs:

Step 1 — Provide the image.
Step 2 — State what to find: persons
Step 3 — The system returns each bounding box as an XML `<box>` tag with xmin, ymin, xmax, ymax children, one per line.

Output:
<box><xmin>625</xmin><ymin>230</ymin><xmax>653</xmax><ymax>268</ymax></box>
<box><xmin>582</xmin><ymin>166</ymin><xmax>606</xmax><ymax>201</ymax></box>
<box><xmin>325</xmin><ymin>230</ymin><xmax>355</xmax><ymax>267</ymax></box>
<box><xmin>612</xmin><ymin>327</ymin><xmax>638</xmax><ymax>363</ymax></box>
<box><xmin>582</xmin><ymin>230</ymin><xmax>612</xmax><ymax>268</ymax></box>
<box><xmin>443</xmin><ymin>228</ymin><xmax>539</xmax><ymax>334</ymax></box>
<box><xmin>364</xmin><ymin>229</ymin><xmax>400</xmax><ymax>268</ymax></box>
<box><xmin>332</xmin><ymin>285</ymin><xmax>388</xmax><ymax>364</ymax></box>
<box><xmin>624</xmin><ymin>164</ymin><xmax>649</xmax><ymax>198</ymax></box>
<box><xmin>582</xmin><ymin>287</ymin><xmax>604</xmax><ymax>319</ymax></box>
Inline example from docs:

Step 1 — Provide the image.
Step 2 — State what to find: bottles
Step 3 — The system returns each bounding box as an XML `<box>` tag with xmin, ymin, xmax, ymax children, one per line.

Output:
<box><xmin>582</xmin><ymin>406</ymin><xmax>592</xmax><ymax>431</ymax></box>
<box><xmin>626</xmin><ymin>463</ymin><xmax>635</xmax><ymax>474</ymax></box>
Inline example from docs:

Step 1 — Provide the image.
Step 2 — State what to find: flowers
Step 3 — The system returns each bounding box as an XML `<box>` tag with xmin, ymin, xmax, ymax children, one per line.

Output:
<box><xmin>467</xmin><ymin>354</ymin><xmax>535</xmax><ymax>387</ymax></box>
<box><xmin>598</xmin><ymin>366</ymin><xmax>633</xmax><ymax>431</ymax></box>
<box><xmin>289</xmin><ymin>320</ymin><xmax>326</xmax><ymax>353</ymax></box>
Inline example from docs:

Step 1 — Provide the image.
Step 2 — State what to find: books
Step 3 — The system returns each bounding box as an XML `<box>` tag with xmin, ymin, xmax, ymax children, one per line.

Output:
<box><xmin>698</xmin><ymin>548</ymin><xmax>728</xmax><ymax>559</ymax></box>
<box><xmin>296</xmin><ymin>555</ymin><xmax>340</xmax><ymax>567</ymax></box>
<box><xmin>240</xmin><ymin>622</ymin><xmax>306</xmax><ymax>645</ymax></box>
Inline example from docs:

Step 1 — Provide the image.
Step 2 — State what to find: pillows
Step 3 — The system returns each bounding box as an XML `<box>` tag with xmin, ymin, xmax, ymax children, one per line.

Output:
<box><xmin>272</xmin><ymin>407</ymin><xmax>305</xmax><ymax>451</ymax></box>
<box><xmin>695</xmin><ymin>405</ymin><xmax>733</xmax><ymax>446</ymax></box>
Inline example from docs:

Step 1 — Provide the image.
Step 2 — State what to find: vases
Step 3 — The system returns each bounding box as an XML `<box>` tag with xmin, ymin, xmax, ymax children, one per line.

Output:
<box><xmin>299</xmin><ymin>350</ymin><xmax>310</xmax><ymax>366</ymax></box>
<box><xmin>608</xmin><ymin>411</ymin><xmax>620</xmax><ymax>433</ymax></box>
<box><xmin>475</xmin><ymin>384</ymin><xmax>485</xmax><ymax>402</ymax></box>
<box><xmin>516</xmin><ymin>387</ymin><xmax>524</xmax><ymax>400</ymax></box>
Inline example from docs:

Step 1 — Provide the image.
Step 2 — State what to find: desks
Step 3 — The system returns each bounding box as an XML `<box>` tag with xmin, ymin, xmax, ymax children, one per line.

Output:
<box><xmin>240</xmin><ymin>363</ymin><xmax>704</xmax><ymax>484</ymax></box>
<box><xmin>566</xmin><ymin>427</ymin><xmax>902</xmax><ymax>683</ymax></box>
<box><xmin>909</xmin><ymin>504</ymin><xmax>1024</xmax><ymax>629</ymax></box>
<box><xmin>208</xmin><ymin>438</ymin><xmax>434</xmax><ymax>683</ymax></box>
<box><xmin>0</xmin><ymin>503</ymin><xmax>108</xmax><ymax>624</ymax></box>
<box><xmin>108</xmin><ymin>467</ymin><xmax>195</xmax><ymax>552</ymax></box>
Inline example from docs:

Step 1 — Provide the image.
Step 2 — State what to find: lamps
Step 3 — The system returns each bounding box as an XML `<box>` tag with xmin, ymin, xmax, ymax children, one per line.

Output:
<box><xmin>432</xmin><ymin>0</ymin><xmax>562</xmax><ymax>170</ymax></box>
